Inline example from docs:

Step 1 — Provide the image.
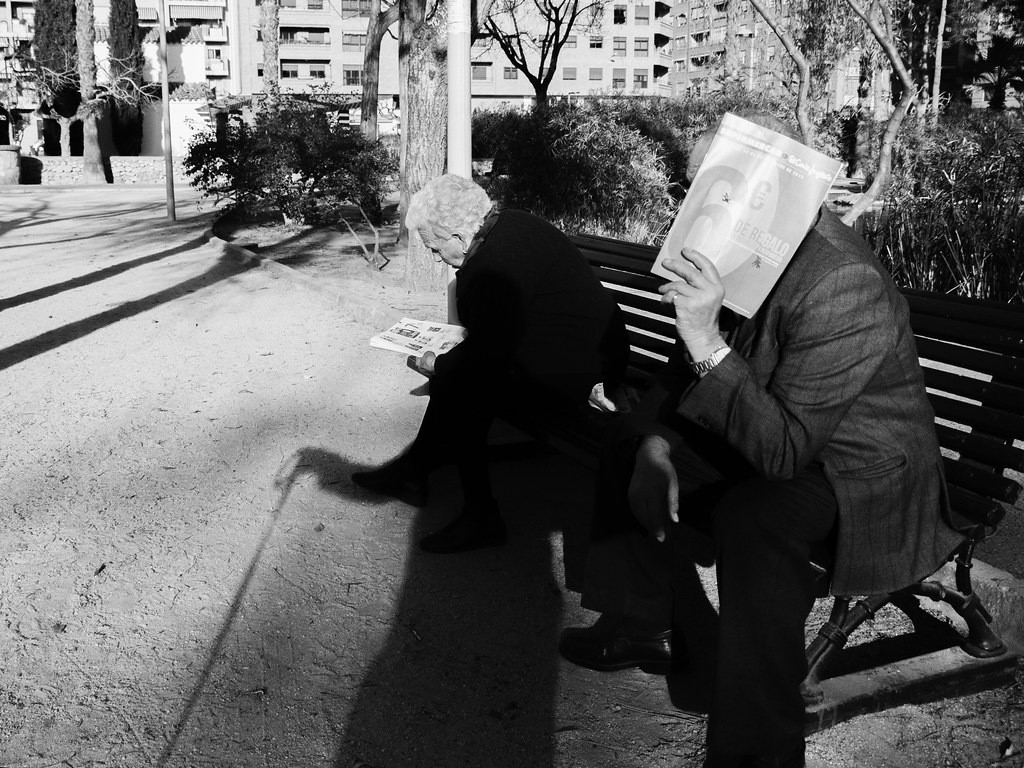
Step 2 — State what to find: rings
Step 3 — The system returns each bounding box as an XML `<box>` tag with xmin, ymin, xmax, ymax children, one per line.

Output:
<box><xmin>672</xmin><ymin>293</ymin><xmax>679</xmax><ymax>303</ymax></box>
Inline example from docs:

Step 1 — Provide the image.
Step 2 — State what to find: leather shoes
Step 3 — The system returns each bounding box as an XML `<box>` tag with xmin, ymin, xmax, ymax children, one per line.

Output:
<box><xmin>564</xmin><ymin>620</ymin><xmax>688</xmax><ymax>675</ymax></box>
<box><xmin>703</xmin><ymin>738</ymin><xmax>807</xmax><ymax>768</ymax></box>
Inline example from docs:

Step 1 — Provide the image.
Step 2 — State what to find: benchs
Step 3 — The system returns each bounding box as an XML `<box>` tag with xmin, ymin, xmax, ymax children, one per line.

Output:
<box><xmin>567</xmin><ymin>233</ymin><xmax>1024</xmax><ymax>705</ymax></box>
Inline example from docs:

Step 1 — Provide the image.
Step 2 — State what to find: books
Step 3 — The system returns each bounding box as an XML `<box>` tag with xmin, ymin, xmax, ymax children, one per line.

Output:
<box><xmin>369</xmin><ymin>317</ymin><xmax>466</xmax><ymax>358</ymax></box>
<box><xmin>649</xmin><ymin>112</ymin><xmax>844</xmax><ymax>321</ymax></box>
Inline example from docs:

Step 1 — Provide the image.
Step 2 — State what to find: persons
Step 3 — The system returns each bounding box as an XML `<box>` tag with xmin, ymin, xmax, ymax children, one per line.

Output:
<box><xmin>560</xmin><ymin>111</ymin><xmax>980</xmax><ymax>768</ymax></box>
<box><xmin>351</xmin><ymin>173</ymin><xmax>631</xmax><ymax>554</ymax></box>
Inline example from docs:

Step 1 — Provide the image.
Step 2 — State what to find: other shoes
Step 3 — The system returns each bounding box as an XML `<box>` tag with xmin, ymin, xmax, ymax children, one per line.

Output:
<box><xmin>419</xmin><ymin>514</ymin><xmax>509</xmax><ymax>554</ymax></box>
<box><xmin>351</xmin><ymin>467</ymin><xmax>427</xmax><ymax>507</ymax></box>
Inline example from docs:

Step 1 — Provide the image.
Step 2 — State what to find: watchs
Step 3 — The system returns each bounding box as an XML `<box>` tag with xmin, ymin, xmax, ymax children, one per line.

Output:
<box><xmin>691</xmin><ymin>347</ymin><xmax>732</xmax><ymax>373</ymax></box>
